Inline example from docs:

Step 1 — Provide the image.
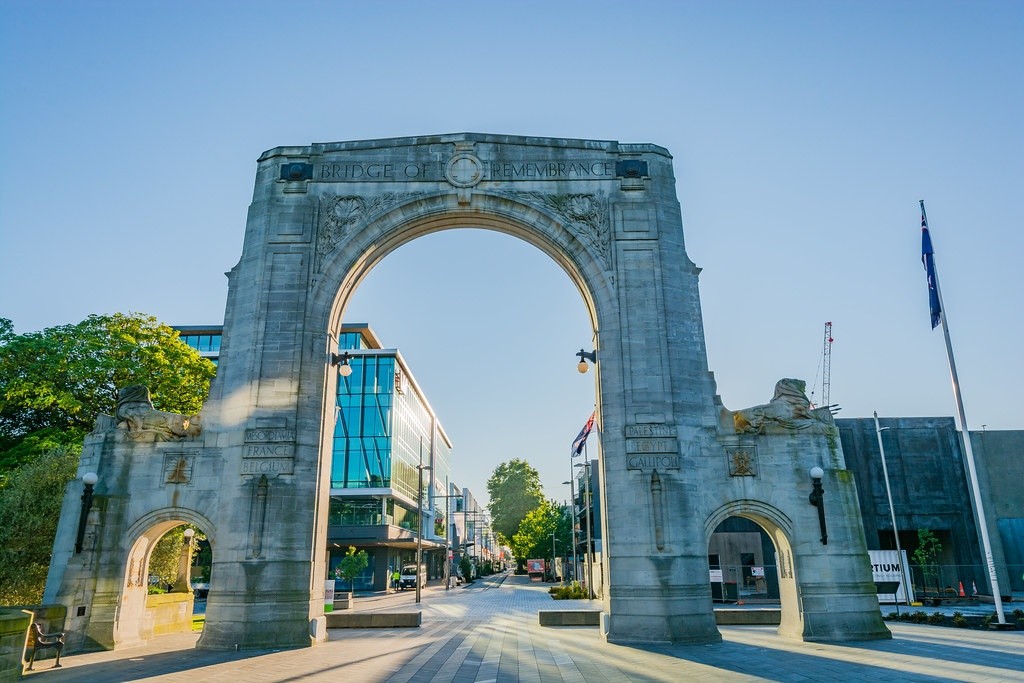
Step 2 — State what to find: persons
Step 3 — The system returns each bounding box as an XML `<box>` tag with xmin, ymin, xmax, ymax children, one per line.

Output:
<box><xmin>404</xmin><ymin>569</ymin><xmax>410</xmax><ymax>575</ymax></box>
<box><xmin>392</xmin><ymin>570</ymin><xmax>400</xmax><ymax>590</ymax></box>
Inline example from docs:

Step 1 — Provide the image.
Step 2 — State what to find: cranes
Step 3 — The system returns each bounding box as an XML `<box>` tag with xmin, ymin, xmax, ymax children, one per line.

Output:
<box><xmin>808</xmin><ymin>320</ymin><xmax>842</xmax><ymax>415</ymax></box>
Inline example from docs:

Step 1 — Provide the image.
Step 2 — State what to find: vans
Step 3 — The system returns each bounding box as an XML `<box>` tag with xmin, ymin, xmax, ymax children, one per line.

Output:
<box><xmin>400</xmin><ymin>566</ymin><xmax>427</xmax><ymax>591</ymax></box>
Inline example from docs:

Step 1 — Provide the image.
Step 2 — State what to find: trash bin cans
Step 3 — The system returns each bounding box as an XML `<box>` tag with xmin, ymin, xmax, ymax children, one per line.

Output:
<box><xmin>724</xmin><ymin>582</ymin><xmax>740</xmax><ymax>599</ymax></box>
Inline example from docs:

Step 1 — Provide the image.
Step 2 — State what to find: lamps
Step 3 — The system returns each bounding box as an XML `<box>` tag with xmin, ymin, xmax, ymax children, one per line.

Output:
<box><xmin>183</xmin><ymin>529</ymin><xmax>194</xmax><ymax>545</ymax></box>
<box><xmin>332</xmin><ymin>351</ymin><xmax>354</xmax><ymax>376</ymax></box>
<box><xmin>575</xmin><ymin>348</ymin><xmax>596</xmax><ymax>373</ymax></box>
<box><xmin>809</xmin><ymin>467</ymin><xmax>829</xmax><ymax>546</ymax></box>
<box><xmin>75</xmin><ymin>472</ymin><xmax>98</xmax><ymax>555</ymax></box>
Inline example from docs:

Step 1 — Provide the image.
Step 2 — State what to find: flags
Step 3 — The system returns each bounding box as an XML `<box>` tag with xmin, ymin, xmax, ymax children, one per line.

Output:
<box><xmin>571</xmin><ymin>411</ymin><xmax>594</xmax><ymax>456</ymax></box>
<box><xmin>919</xmin><ymin>208</ymin><xmax>943</xmax><ymax>332</ymax></box>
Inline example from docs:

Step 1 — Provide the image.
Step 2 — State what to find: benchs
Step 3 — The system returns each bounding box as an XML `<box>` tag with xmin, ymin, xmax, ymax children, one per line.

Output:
<box><xmin>25</xmin><ymin>623</ymin><xmax>65</xmax><ymax>671</ymax></box>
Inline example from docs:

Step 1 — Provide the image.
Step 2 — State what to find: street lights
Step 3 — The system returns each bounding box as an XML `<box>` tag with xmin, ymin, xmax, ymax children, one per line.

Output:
<box><xmin>574</xmin><ymin>462</ymin><xmax>594</xmax><ymax>600</ymax></box>
<box><xmin>453</xmin><ymin>510</ymin><xmax>478</xmax><ymax>553</ymax></box>
<box><xmin>431</xmin><ymin>495</ymin><xmax>464</xmax><ymax>589</ymax></box>
<box><xmin>876</xmin><ymin>426</ymin><xmax>911</xmax><ymax>606</ymax></box>
<box><xmin>548</xmin><ymin>534</ymin><xmax>561</xmax><ymax>582</ymax></box>
<box><xmin>417</xmin><ymin>464</ymin><xmax>434</xmax><ymax>602</ymax></box>
<box><xmin>563</xmin><ymin>481</ymin><xmax>577</xmax><ymax>581</ymax></box>
<box><xmin>466</xmin><ymin>520</ymin><xmax>494</xmax><ymax>576</ymax></box>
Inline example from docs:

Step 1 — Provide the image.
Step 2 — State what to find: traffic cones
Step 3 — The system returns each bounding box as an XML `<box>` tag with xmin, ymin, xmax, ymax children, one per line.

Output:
<box><xmin>959</xmin><ymin>581</ymin><xmax>966</xmax><ymax>597</ymax></box>
